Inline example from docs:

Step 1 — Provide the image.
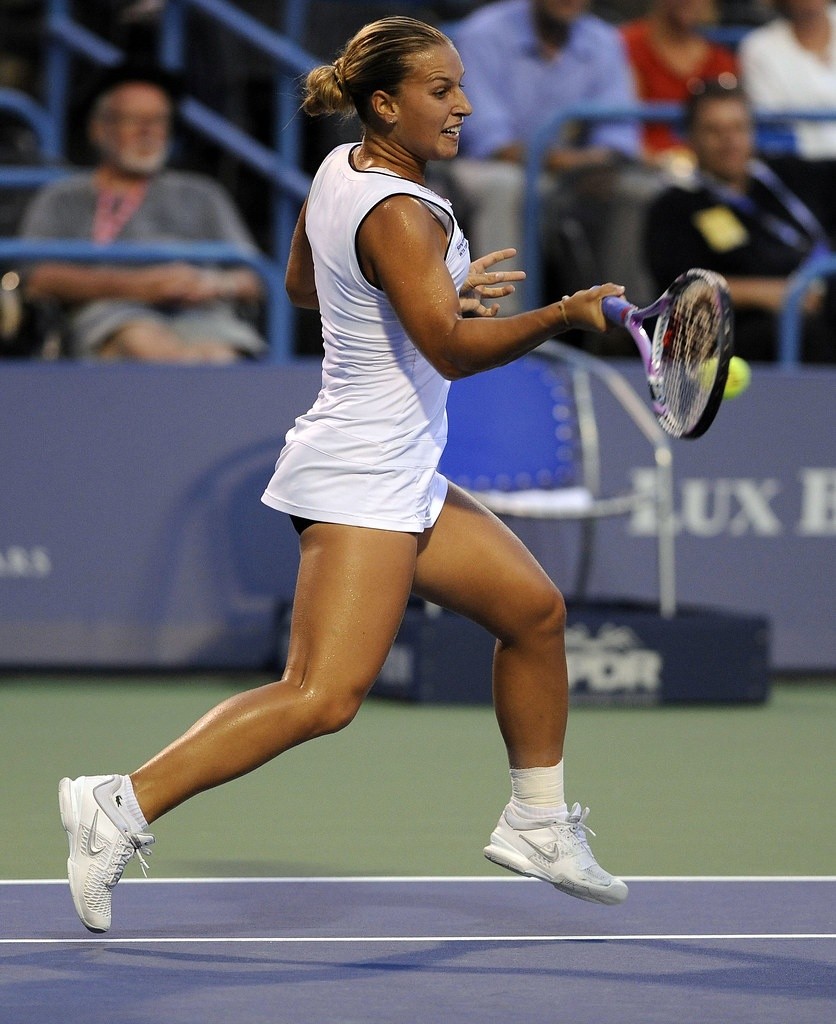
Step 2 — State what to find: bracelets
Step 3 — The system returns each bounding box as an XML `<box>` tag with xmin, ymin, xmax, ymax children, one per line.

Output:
<box><xmin>559</xmin><ymin>295</ymin><xmax>571</xmax><ymax>327</ymax></box>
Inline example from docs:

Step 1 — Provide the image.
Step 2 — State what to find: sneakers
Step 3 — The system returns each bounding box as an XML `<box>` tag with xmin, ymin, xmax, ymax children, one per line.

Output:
<box><xmin>482</xmin><ymin>799</ymin><xmax>629</xmax><ymax>905</ymax></box>
<box><xmin>59</xmin><ymin>775</ymin><xmax>155</xmax><ymax>933</ymax></box>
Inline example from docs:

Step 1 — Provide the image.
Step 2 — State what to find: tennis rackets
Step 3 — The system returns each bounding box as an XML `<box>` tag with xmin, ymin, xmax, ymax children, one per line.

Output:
<box><xmin>604</xmin><ymin>265</ymin><xmax>735</xmax><ymax>440</ymax></box>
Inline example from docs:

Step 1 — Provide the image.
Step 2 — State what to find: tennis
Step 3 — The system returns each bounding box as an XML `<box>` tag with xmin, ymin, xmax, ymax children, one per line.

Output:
<box><xmin>703</xmin><ymin>354</ymin><xmax>755</xmax><ymax>401</ymax></box>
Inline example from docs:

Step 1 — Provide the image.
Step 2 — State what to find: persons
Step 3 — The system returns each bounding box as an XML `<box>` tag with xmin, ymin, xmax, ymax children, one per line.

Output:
<box><xmin>454</xmin><ymin>0</ymin><xmax>836</xmax><ymax>365</ymax></box>
<box><xmin>20</xmin><ymin>55</ymin><xmax>267</xmax><ymax>361</ymax></box>
<box><xmin>58</xmin><ymin>16</ymin><xmax>628</xmax><ymax>934</ymax></box>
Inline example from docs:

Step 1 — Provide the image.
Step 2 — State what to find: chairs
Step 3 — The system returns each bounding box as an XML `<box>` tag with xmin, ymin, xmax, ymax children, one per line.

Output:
<box><xmin>438</xmin><ymin>342</ymin><xmax>677</xmax><ymax>617</ymax></box>
